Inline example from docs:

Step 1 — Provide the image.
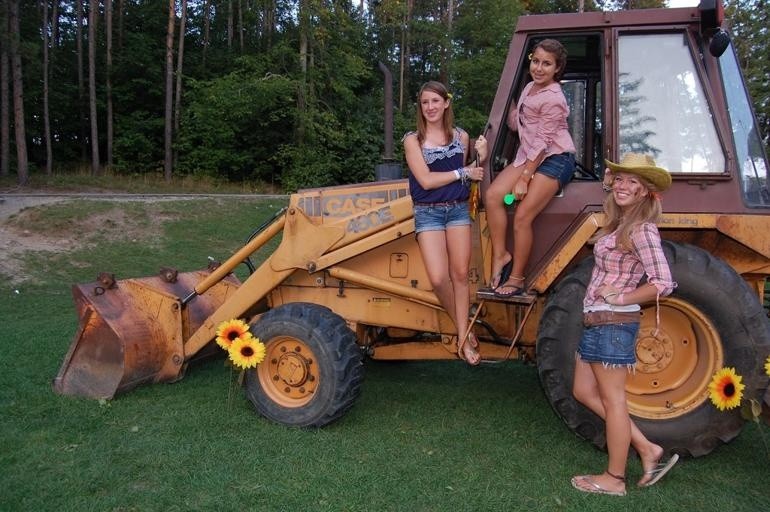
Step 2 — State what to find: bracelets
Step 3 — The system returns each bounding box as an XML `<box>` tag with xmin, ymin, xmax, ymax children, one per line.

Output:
<box><xmin>524</xmin><ymin>169</ymin><xmax>536</xmax><ymax>180</ymax></box>
<box><xmin>603</xmin><ymin>293</ymin><xmax>616</xmax><ymax>302</ymax></box>
<box><xmin>452</xmin><ymin>167</ymin><xmax>460</xmax><ymax>179</ymax></box>
<box><xmin>619</xmin><ymin>292</ymin><xmax>623</xmax><ymax>304</ymax></box>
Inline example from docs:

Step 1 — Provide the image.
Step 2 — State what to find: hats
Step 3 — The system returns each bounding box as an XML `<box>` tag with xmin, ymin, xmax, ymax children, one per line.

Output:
<box><xmin>605</xmin><ymin>152</ymin><xmax>671</xmax><ymax>190</ymax></box>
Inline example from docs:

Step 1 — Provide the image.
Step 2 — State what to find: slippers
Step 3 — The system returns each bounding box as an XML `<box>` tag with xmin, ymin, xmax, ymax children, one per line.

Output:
<box><xmin>493</xmin><ymin>285</ymin><xmax>526</xmax><ymax>298</ymax></box>
<box><xmin>490</xmin><ymin>259</ymin><xmax>513</xmax><ymax>290</ymax></box>
<box><xmin>637</xmin><ymin>453</ymin><xmax>679</xmax><ymax>487</ymax></box>
<box><xmin>458</xmin><ymin>322</ymin><xmax>481</xmax><ymax>366</ymax></box>
<box><xmin>571</xmin><ymin>475</ymin><xmax>627</xmax><ymax>496</ymax></box>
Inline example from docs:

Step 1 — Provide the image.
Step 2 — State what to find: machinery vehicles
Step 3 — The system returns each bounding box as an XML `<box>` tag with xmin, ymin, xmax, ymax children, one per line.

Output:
<box><xmin>49</xmin><ymin>0</ymin><xmax>770</xmax><ymax>464</ymax></box>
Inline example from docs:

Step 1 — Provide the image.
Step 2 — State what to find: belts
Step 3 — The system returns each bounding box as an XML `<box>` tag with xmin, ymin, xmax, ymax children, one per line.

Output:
<box><xmin>413</xmin><ymin>199</ymin><xmax>467</xmax><ymax>206</ymax></box>
<box><xmin>583</xmin><ymin>310</ymin><xmax>642</xmax><ymax>326</ymax></box>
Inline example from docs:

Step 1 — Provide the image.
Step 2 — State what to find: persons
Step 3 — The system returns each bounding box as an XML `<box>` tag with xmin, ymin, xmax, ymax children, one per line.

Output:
<box><xmin>484</xmin><ymin>39</ymin><xmax>580</xmax><ymax>296</ymax></box>
<box><xmin>404</xmin><ymin>80</ymin><xmax>493</xmax><ymax>365</ymax></box>
<box><xmin>573</xmin><ymin>150</ymin><xmax>684</xmax><ymax>497</ymax></box>
